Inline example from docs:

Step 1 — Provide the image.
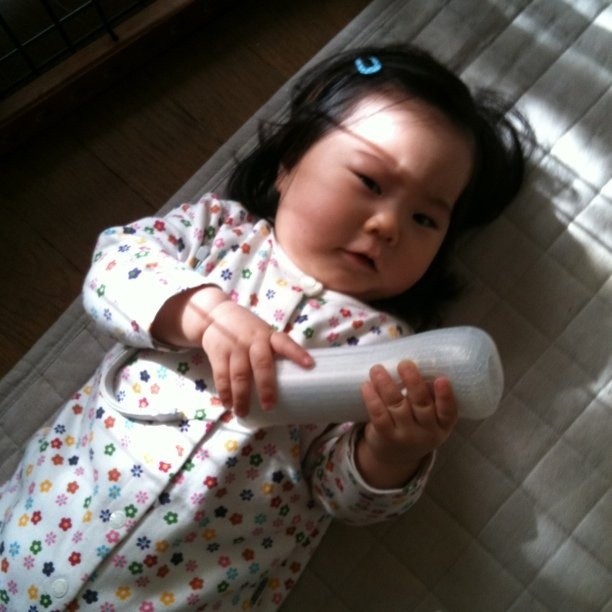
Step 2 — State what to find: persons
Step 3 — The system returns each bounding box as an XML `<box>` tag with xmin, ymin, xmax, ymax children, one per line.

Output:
<box><xmin>0</xmin><ymin>38</ymin><xmax>539</xmax><ymax>612</ymax></box>
